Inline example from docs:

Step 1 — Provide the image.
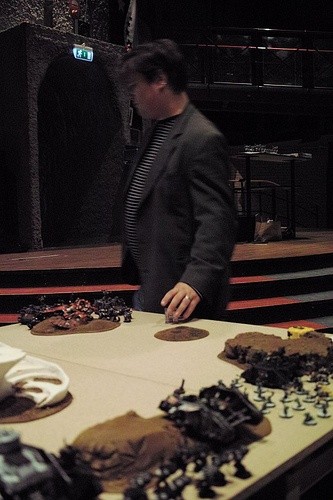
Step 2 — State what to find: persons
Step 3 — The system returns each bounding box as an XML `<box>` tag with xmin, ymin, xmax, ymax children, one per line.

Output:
<box><xmin>118</xmin><ymin>37</ymin><xmax>241</xmax><ymax>324</ymax></box>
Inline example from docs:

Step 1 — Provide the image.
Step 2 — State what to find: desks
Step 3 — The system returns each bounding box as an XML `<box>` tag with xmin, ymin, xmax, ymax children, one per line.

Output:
<box><xmin>231</xmin><ymin>152</ymin><xmax>312</xmax><ymax>242</ymax></box>
<box><xmin>0</xmin><ymin>310</ymin><xmax>333</xmax><ymax>500</ymax></box>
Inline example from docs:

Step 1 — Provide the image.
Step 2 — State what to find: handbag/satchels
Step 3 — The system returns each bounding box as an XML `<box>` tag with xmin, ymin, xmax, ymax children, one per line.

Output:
<box><xmin>251</xmin><ymin>214</ymin><xmax>282</xmax><ymax>244</ymax></box>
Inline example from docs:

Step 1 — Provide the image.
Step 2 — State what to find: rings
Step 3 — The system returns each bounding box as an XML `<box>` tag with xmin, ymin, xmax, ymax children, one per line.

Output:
<box><xmin>185</xmin><ymin>295</ymin><xmax>192</xmax><ymax>302</ymax></box>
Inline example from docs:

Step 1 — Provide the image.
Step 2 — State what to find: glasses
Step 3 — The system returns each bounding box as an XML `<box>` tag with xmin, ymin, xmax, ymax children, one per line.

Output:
<box><xmin>127</xmin><ymin>77</ymin><xmax>145</xmax><ymax>96</ymax></box>
